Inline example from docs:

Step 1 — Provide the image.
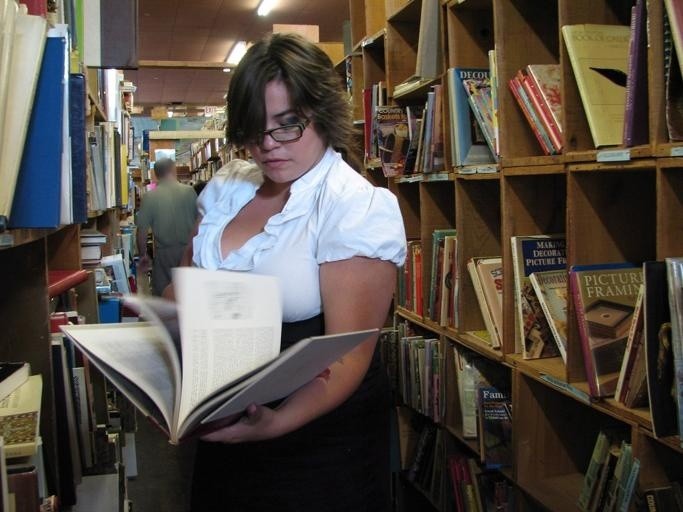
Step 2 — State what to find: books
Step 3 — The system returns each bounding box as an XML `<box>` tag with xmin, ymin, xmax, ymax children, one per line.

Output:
<box><xmin>192</xmin><ymin>122</ymin><xmax>253</xmax><ymax>186</ymax></box>
<box><xmin>58</xmin><ymin>267</ymin><xmax>380</xmax><ymax>446</ymax></box>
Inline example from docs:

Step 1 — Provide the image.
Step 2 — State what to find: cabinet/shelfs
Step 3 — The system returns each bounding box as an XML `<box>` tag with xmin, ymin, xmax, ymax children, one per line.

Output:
<box><xmin>130</xmin><ymin>132</ymin><xmax>254</xmax><ymax>183</ymax></box>
<box><xmin>0</xmin><ymin>0</ymin><xmax>136</xmax><ymax>512</ymax></box>
<box><xmin>319</xmin><ymin>0</ymin><xmax>683</xmax><ymax>511</ymax></box>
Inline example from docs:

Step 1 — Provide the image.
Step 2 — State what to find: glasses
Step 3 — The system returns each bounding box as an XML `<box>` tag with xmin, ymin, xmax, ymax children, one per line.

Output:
<box><xmin>244</xmin><ymin>114</ymin><xmax>322</xmax><ymax>145</ymax></box>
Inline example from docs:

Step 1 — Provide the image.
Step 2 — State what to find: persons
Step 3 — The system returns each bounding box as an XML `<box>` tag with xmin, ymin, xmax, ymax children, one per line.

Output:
<box><xmin>136</xmin><ymin>157</ymin><xmax>198</xmax><ymax>296</ymax></box>
<box><xmin>161</xmin><ymin>33</ymin><xmax>408</xmax><ymax>512</ymax></box>
